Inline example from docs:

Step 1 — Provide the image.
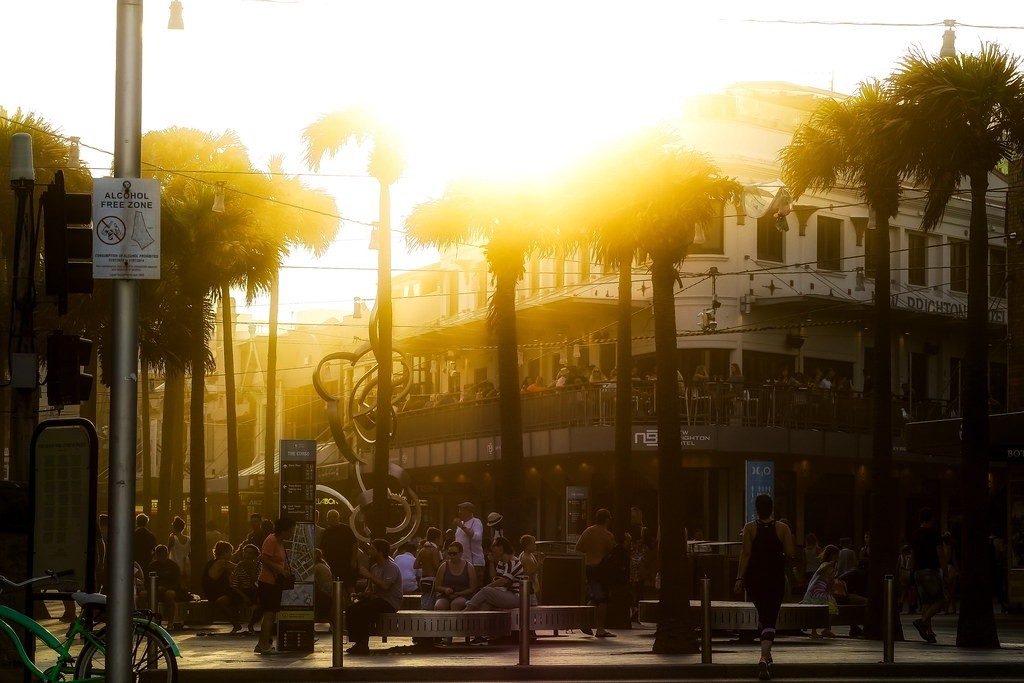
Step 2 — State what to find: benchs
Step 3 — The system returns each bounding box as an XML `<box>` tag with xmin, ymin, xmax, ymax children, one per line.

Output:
<box><xmin>158</xmin><ymin>599</ymin><xmax>250</xmax><ymax>624</ymax></box>
<box><xmin>371</xmin><ymin>603</ymin><xmax>827</xmax><ymax>646</ymax></box>
<box><xmin>494</xmin><ymin>603</ymin><xmax>792</xmax><ymax>632</ymax></box>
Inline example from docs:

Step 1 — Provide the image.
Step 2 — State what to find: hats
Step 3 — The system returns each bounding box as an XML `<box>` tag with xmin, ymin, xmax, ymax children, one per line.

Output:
<box><xmin>487</xmin><ymin>512</ymin><xmax>503</xmax><ymax>526</ymax></box>
<box><xmin>457</xmin><ymin>502</ymin><xmax>475</xmax><ymax>512</ymax></box>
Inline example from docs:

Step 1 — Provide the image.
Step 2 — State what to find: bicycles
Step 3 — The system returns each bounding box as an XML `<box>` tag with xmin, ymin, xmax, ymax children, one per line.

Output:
<box><xmin>0</xmin><ymin>567</ymin><xmax>183</xmax><ymax>683</ymax></box>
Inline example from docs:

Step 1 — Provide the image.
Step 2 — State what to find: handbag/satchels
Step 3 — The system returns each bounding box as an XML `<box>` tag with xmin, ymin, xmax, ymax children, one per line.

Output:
<box><xmin>350</xmin><ymin>593</ymin><xmax>381</xmax><ymax>613</ymax></box>
<box><xmin>276</xmin><ymin>573</ymin><xmax>296</xmax><ymax>590</ymax></box>
<box><xmin>600</xmin><ymin>540</ymin><xmax>631</xmax><ymax>579</ymax></box>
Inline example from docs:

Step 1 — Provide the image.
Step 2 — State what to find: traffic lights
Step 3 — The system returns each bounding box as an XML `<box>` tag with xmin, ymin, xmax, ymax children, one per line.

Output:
<box><xmin>44</xmin><ymin>334</ymin><xmax>93</xmax><ymax>406</ymax></box>
<box><xmin>43</xmin><ymin>169</ymin><xmax>94</xmax><ymax>306</ymax></box>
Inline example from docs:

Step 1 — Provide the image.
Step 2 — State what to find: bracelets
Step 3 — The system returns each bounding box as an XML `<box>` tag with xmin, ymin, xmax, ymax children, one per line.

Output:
<box><xmin>736</xmin><ymin>577</ymin><xmax>744</xmax><ymax>581</ymax></box>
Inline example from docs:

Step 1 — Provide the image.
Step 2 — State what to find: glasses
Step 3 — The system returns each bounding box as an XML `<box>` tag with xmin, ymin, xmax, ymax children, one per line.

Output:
<box><xmin>448</xmin><ymin>551</ymin><xmax>461</xmax><ymax>555</ymax></box>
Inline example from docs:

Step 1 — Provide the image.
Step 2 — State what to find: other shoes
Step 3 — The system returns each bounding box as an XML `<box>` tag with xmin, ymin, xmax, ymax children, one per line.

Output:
<box><xmin>580</xmin><ymin>624</ymin><xmax>594</xmax><ymax>635</ymax></box>
<box><xmin>912</xmin><ymin>619</ymin><xmax>937</xmax><ymax>643</ymax></box>
<box><xmin>821</xmin><ymin>630</ymin><xmax>835</xmax><ymax>637</ymax></box>
<box><xmin>254</xmin><ymin>643</ymin><xmax>278</xmax><ymax>655</ymax></box>
<box><xmin>346</xmin><ymin>642</ymin><xmax>370</xmax><ymax>653</ymax></box>
<box><xmin>759</xmin><ymin>652</ymin><xmax>773</xmax><ymax>680</ymax></box>
<box><xmin>596</xmin><ymin>631</ymin><xmax>616</xmax><ymax>637</ymax></box>
<box><xmin>470</xmin><ymin>636</ymin><xmax>488</xmax><ymax>646</ymax></box>
<box><xmin>810</xmin><ymin>633</ymin><xmax>823</xmax><ymax>639</ymax></box>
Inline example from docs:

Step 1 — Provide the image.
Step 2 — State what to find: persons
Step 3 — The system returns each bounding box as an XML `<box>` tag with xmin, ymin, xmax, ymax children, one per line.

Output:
<box><xmin>484</xmin><ymin>363</ymin><xmax>931</xmax><ymax>434</ymax></box>
<box><xmin>913</xmin><ymin>508</ymin><xmax>951</xmax><ymax>644</ymax></box>
<box><xmin>734</xmin><ymin>494</ymin><xmax>795</xmax><ymax>682</ymax></box>
<box><xmin>576</xmin><ymin>507</ymin><xmax>649</xmax><ymax>637</ymax></box>
<box><xmin>312</xmin><ymin>502</ymin><xmax>546</xmax><ymax>657</ymax></box>
<box><xmin>57</xmin><ymin>510</ymin><xmax>294</xmax><ymax>655</ymax></box>
<box><xmin>896</xmin><ymin>544</ymin><xmax>957</xmax><ymax>615</ymax></box>
<box><xmin>803</xmin><ymin>531</ymin><xmax>872</xmax><ymax>640</ymax></box>
